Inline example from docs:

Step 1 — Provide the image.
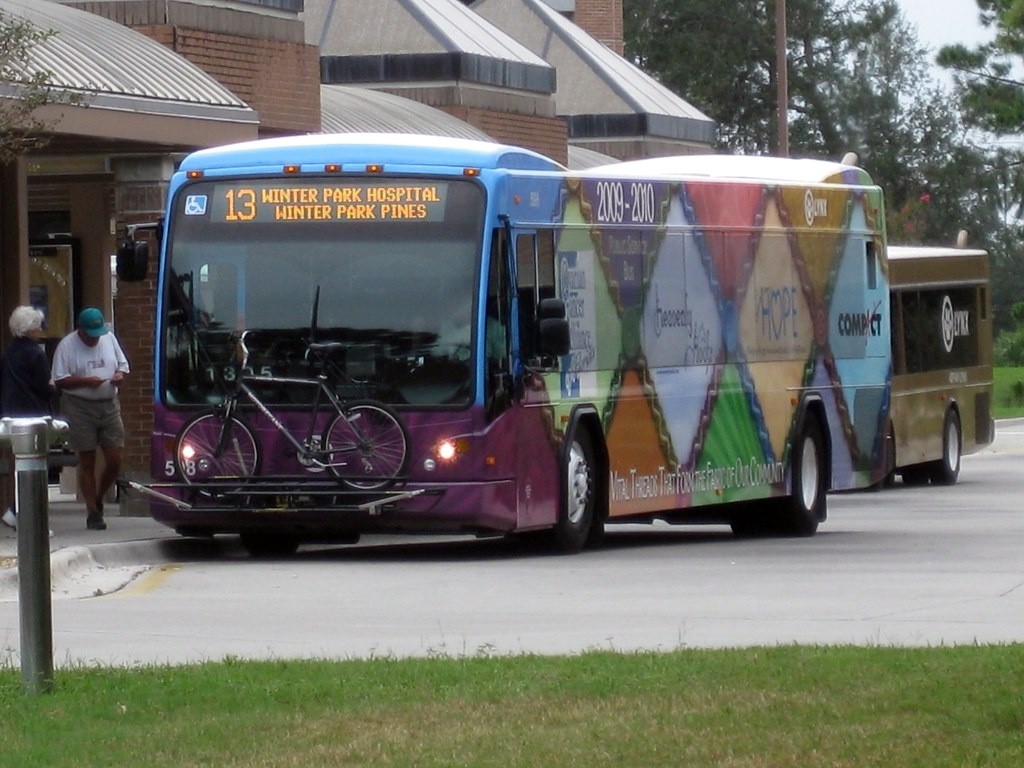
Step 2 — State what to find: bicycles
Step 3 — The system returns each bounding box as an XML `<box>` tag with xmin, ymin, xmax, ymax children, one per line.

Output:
<box><xmin>174</xmin><ymin>326</ymin><xmax>413</xmax><ymax>501</ymax></box>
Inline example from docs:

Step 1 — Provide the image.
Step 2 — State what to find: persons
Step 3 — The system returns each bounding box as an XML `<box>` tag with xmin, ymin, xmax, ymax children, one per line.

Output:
<box><xmin>431</xmin><ymin>267</ymin><xmax>505</xmax><ymax>359</ymax></box>
<box><xmin>0</xmin><ymin>305</ymin><xmax>53</xmax><ymax>539</ymax></box>
<box><xmin>51</xmin><ymin>308</ymin><xmax>130</xmax><ymax>530</ymax></box>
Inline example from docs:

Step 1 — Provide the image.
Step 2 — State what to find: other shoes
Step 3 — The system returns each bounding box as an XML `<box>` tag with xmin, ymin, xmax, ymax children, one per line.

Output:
<box><xmin>2</xmin><ymin>507</ymin><xmax>17</xmax><ymax>531</ymax></box>
<box><xmin>87</xmin><ymin>516</ymin><xmax>106</xmax><ymax>530</ymax></box>
<box><xmin>49</xmin><ymin>530</ymin><xmax>54</xmax><ymax>537</ymax></box>
<box><xmin>97</xmin><ymin>503</ymin><xmax>103</xmax><ymax>517</ymax></box>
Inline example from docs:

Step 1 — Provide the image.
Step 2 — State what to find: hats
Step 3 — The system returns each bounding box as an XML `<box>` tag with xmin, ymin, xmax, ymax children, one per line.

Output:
<box><xmin>79</xmin><ymin>307</ymin><xmax>109</xmax><ymax>337</ymax></box>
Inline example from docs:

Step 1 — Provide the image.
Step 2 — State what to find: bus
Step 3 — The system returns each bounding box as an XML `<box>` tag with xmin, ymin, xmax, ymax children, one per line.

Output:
<box><xmin>115</xmin><ymin>134</ymin><xmax>891</xmax><ymax>554</ymax></box>
<box><xmin>885</xmin><ymin>244</ymin><xmax>995</xmax><ymax>488</ymax></box>
